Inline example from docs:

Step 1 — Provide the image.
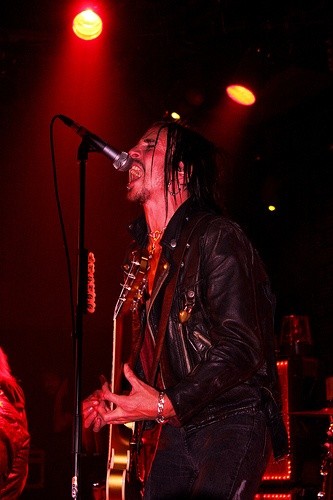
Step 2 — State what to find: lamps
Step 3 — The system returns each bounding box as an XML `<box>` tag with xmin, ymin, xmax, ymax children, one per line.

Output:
<box><xmin>226</xmin><ymin>48</ymin><xmax>272</xmax><ymax>106</ymax></box>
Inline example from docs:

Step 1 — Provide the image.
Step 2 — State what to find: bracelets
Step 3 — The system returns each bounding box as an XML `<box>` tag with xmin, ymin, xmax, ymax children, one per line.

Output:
<box><xmin>156</xmin><ymin>391</ymin><xmax>166</xmax><ymax>423</ymax></box>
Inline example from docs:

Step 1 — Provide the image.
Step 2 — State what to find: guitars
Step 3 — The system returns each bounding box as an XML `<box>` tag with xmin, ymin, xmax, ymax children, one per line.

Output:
<box><xmin>105</xmin><ymin>250</ymin><xmax>150</xmax><ymax>500</ymax></box>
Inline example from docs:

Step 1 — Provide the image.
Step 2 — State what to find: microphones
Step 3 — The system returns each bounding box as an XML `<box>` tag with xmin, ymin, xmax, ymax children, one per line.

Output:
<box><xmin>57</xmin><ymin>115</ymin><xmax>131</xmax><ymax>171</ymax></box>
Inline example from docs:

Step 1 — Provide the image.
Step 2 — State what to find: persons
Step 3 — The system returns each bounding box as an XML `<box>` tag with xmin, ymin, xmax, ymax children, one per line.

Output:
<box><xmin>78</xmin><ymin>121</ymin><xmax>282</xmax><ymax>500</ymax></box>
<box><xmin>0</xmin><ymin>343</ymin><xmax>30</xmax><ymax>500</ymax></box>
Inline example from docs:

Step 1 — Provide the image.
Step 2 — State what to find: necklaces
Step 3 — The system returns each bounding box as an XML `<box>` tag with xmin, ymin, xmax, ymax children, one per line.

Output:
<box><xmin>147</xmin><ymin>225</ymin><xmax>168</xmax><ymax>241</ymax></box>
